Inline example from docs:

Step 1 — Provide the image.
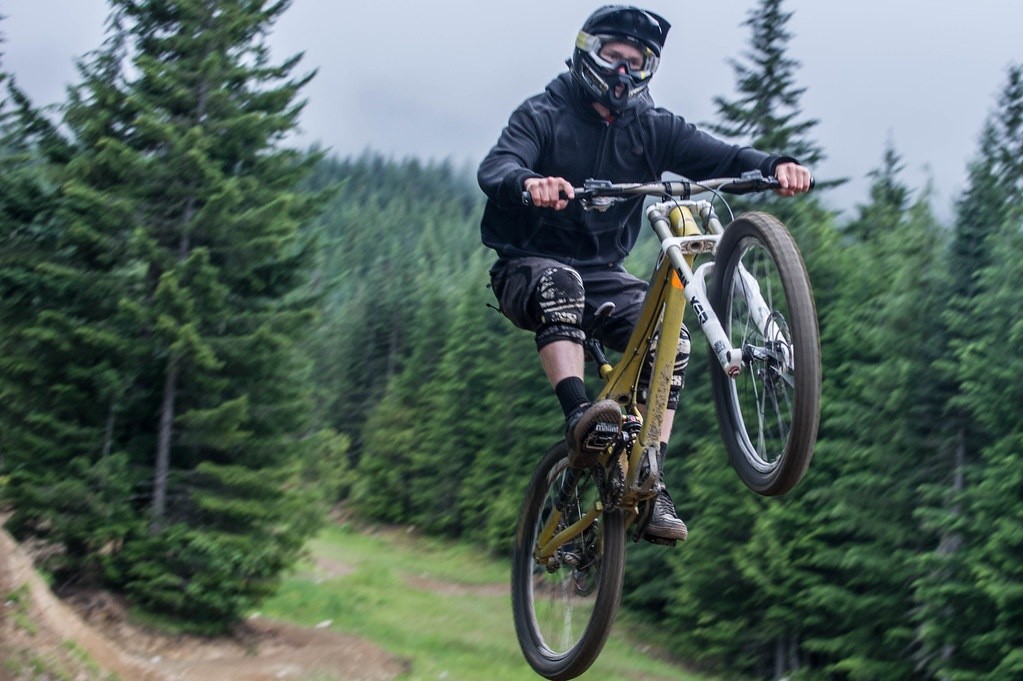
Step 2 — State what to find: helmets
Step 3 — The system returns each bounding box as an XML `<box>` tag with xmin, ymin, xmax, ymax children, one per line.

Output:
<box><xmin>570</xmin><ymin>4</ymin><xmax>670</xmax><ymax>111</ymax></box>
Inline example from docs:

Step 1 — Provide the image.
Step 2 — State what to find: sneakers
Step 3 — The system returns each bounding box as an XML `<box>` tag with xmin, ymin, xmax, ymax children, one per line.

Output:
<box><xmin>561</xmin><ymin>400</ymin><xmax>623</xmax><ymax>467</ymax></box>
<box><xmin>637</xmin><ymin>465</ymin><xmax>687</xmax><ymax>541</ymax></box>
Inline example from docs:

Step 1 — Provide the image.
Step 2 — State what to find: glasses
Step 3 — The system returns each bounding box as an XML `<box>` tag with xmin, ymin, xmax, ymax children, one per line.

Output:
<box><xmin>591</xmin><ymin>36</ymin><xmax>657</xmax><ymax>79</ymax></box>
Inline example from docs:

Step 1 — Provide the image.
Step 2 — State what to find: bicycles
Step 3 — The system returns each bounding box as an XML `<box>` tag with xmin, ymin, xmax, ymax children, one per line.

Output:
<box><xmin>512</xmin><ymin>172</ymin><xmax>818</xmax><ymax>680</ymax></box>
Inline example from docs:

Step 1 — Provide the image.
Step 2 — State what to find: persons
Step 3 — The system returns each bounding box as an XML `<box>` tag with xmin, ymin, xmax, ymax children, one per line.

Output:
<box><xmin>478</xmin><ymin>5</ymin><xmax>811</xmax><ymax>541</ymax></box>
